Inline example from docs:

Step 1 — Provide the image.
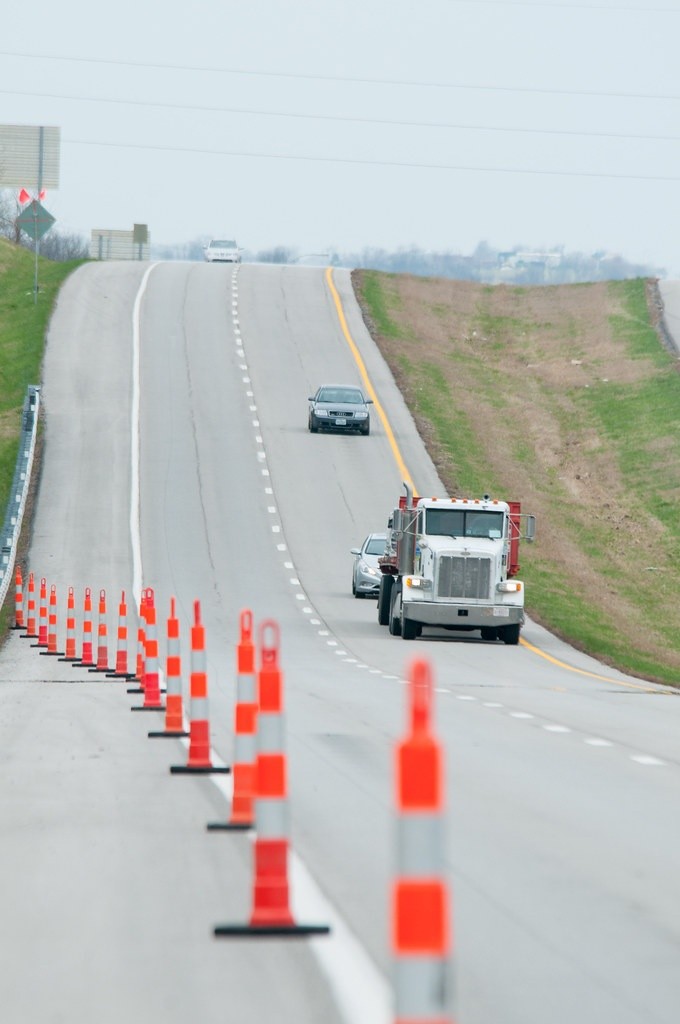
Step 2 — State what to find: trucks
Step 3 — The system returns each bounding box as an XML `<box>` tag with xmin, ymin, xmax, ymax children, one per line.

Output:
<box><xmin>375</xmin><ymin>481</ymin><xmax>538</xmax><ymax>646</ymax></box>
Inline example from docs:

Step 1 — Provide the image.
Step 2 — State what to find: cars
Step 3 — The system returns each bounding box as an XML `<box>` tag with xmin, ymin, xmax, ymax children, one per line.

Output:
<box><xmin>350</xmin><ymin>534</ymin><xmax>399</xmax><ymax>599</ymax></box>
<box><xmin>307</xmin><ymin>385</ymin><xmax>375</xmax><ymax>436</ymax></box>
<box><xmin>202</xmin><ymin>238</ymin><xmax>242</xmax><ymax>263</ymax></box>
